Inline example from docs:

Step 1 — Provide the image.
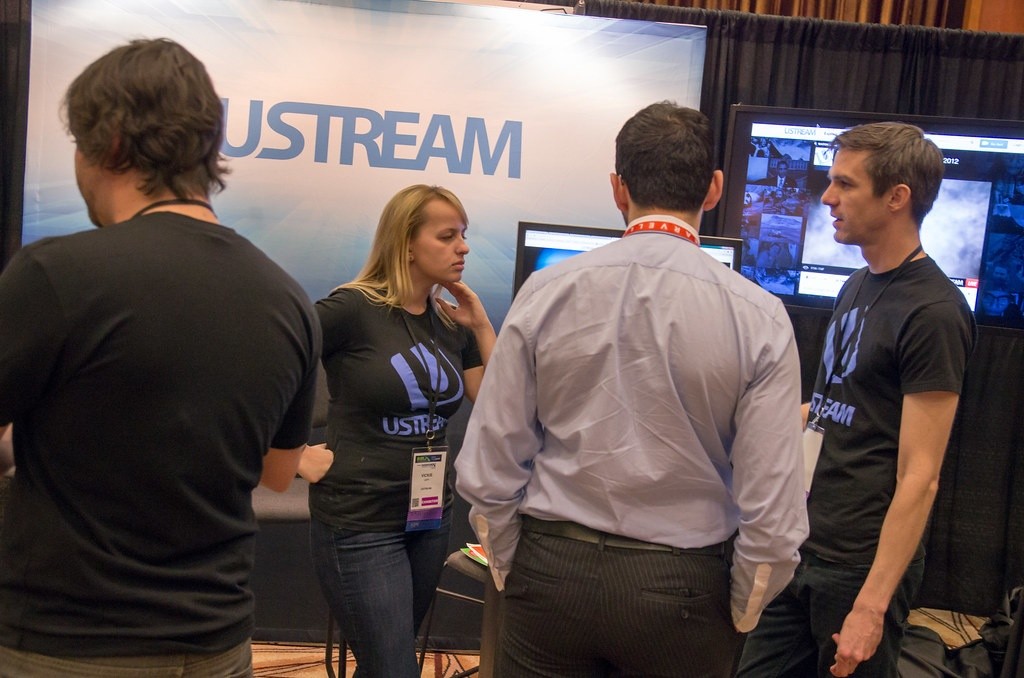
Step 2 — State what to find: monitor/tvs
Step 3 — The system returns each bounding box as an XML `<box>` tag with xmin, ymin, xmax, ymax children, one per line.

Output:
<box><xmin>717</xmin><ymin>104</ymin><xmax>1024</xmax><ymax>335</ymax></box>
<box><xmin>512</xmin><ymin>221</ymin><xmax>743</xmax><ymax>303</ymax></box>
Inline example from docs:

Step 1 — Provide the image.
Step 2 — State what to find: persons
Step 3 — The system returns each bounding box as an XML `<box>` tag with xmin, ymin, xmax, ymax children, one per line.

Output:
<box><xmin>297</xmin><ymin>184</ymin><xmax>498</xmax><ymax>678</ymax></box>
<box><xmin>742</xmin><ymin>136</ymin><xmax>808</xmax><ymax>294</ymax></box>
<box><xmin>454</xmin><ymin>101</ymin><xmax>811</xmax><ymax>678</ymax></box>
<box><xmin>735</xmin><ymin>122</ymin><xmax>978</xmax><ymax>678</ymax></box>
<box><xmin>980</xmin><ymin>152</ymin><xmax>1024</xmax><ymax>316</ymax></box>
<box><xmin>0</xmin><ymin>37</ymin><xmax>322</xmax><ymax>678</ymax></box>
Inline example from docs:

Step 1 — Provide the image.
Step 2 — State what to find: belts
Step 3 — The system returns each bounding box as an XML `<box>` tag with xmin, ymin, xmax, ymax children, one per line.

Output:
<box><xmin>521</xmin><ymin>514</ymin><xmax>732</xmax><ymax>556</ymax></box>
